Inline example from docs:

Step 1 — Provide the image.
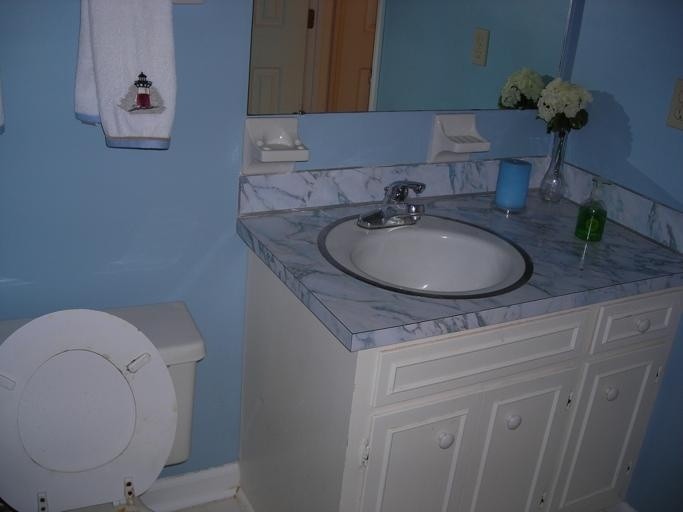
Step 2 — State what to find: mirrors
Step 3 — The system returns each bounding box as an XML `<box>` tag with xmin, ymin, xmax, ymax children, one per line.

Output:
<box><xmin>245</xmin><ymin>0</ymin><xmax>574</xmax><ymax>116</ymax></box>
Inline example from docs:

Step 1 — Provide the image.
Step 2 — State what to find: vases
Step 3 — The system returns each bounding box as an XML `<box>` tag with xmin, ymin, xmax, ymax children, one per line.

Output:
<box><xmin>538</xmin><ymin>127</ymin><xmax>573</xmax><ymax>203</ymax></box>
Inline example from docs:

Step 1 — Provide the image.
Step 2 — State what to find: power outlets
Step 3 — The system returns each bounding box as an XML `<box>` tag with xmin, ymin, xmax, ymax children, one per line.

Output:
<box><xmin>470</xmin><ymin>25</ymin><xmax>492</xmax><ymax>68</ymax></box>
<box><xmin>664</xmin><ymin>79</ymin><xmax>682</xmax><ymax>132</ymax></box>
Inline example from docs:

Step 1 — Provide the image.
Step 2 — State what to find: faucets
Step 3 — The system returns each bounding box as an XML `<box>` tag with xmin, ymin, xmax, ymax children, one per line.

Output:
<box><xmin>357</xmin><ymin>178</ymin><xmax>426</xmax><ymax>229</ymax></box>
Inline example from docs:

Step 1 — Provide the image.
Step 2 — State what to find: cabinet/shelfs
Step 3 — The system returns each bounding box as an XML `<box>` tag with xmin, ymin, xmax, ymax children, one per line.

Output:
<box><xmin>234</xmin><ymin>238</ymin><xmax>596</xmax><ymax>512</ymax></box>
<box><xmin>542</xmin><ymin>270</ymin><xmax>682</xmax><ymax>511</ymax></box>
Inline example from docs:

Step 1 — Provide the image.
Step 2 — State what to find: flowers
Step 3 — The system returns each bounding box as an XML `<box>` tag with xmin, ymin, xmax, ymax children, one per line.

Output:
<box><xmin>496</xmin><ymin>66</ymin><xmax>549</xmax><ymax>113</ymax></box>
<box><xmin>529</xmin><ymin>75</ymin><xmax>599</xmax><ymax>182</ymax></box>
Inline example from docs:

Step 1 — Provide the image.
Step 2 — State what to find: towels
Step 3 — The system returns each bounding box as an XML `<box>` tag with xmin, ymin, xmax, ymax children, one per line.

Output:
<box><xmin>70</xmin><ymin>0</ymin><xmax>178</xmax><ymax>150</ymax></box>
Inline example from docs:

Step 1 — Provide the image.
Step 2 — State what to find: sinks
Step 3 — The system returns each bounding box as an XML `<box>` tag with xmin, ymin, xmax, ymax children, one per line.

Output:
<box><xmin>315</xmin><ymin>211</ymin><xmax>535</xmax><ymax>301</ymax></box>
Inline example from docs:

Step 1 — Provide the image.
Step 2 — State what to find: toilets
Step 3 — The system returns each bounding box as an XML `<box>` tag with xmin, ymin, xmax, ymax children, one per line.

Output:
<box><xmin>0</xmin><ymin>300</ymin><xmax>208</xmax><ymax>512</ymax></box>
